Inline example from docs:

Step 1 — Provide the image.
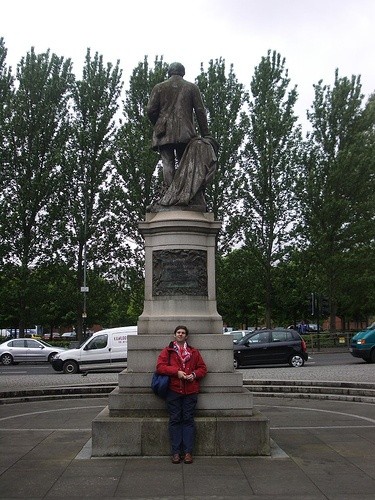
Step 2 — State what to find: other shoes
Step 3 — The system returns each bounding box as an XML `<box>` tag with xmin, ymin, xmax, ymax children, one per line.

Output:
<box><xmin>172</xmin><ymin>451</ymin><xmax>192</xmax><ymax>464</ymax></box>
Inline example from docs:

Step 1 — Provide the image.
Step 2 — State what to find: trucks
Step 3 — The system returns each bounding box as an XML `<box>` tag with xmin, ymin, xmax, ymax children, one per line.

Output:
<box><xmin>51</xmin><ymin>326</ymin><xmax>138</xmax><ymax>373</ymax></box>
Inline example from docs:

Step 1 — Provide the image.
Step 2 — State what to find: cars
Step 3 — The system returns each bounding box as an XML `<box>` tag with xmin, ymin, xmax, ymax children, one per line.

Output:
<box><xmin>0</xmin><ymin>337</ymin><xmax>68</xmax><ymax>366</ymax></box>
<box><xmin>223</xmin><ymin>323</ymin><xmax>322</xmax><ymax>343</ymax></box>
<box><xmin>348</xmin><ymin>321</ymin><xmax>375</xmax><ymax>364</ymax></box>
<box><xmin>42</xmin><ymin>331</ymin><xmax>75</xmax><ymax>340</ymax></box>
<box><xmin>232</xmin><ymin>328</ymin><xmax>309</xmax><ymax>368</ymax></box>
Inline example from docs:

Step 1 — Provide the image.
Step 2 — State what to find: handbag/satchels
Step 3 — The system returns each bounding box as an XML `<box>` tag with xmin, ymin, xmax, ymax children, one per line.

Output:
<box><xmin>150</xmin><ymin>352</ymin><xmax>170</xmax><ymax>399</ymax></box>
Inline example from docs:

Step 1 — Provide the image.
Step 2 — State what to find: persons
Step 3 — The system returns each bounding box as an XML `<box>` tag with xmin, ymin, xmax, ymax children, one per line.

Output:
<box><xmin>156</xmin><ymin>325</ymin><xmax>208</xmax><ymax>464</ymax></box>
<box><xmin>146</xmin><ymin>63</ymin><xmax>209</xmax><ymax>206</ymax></box>
